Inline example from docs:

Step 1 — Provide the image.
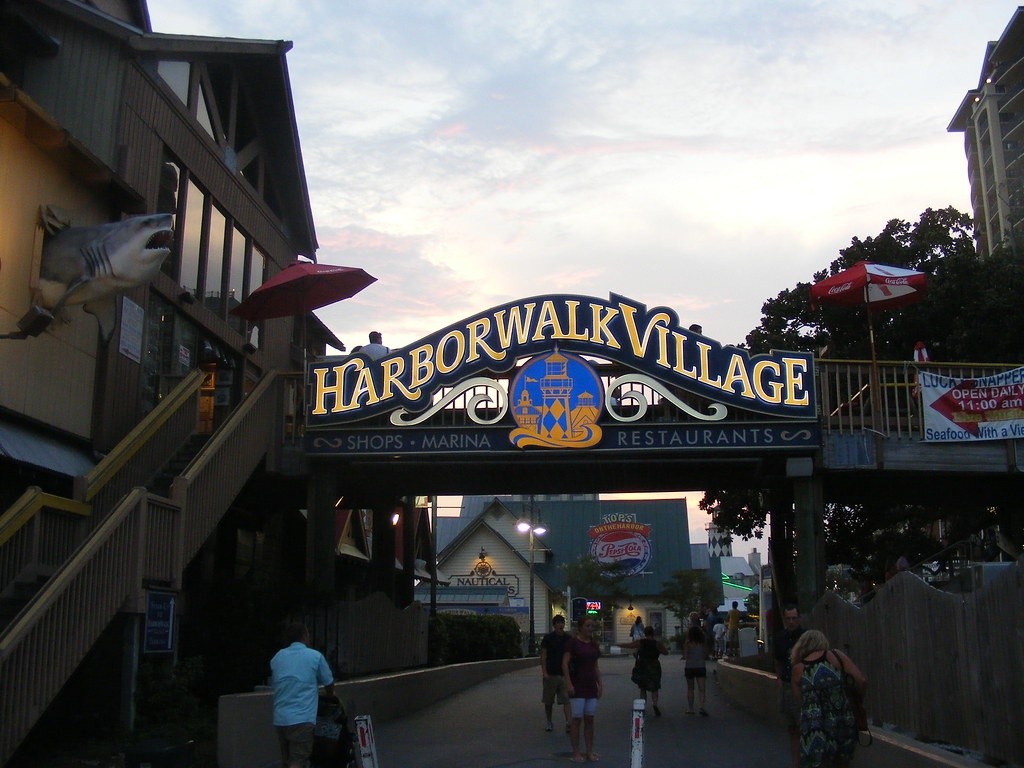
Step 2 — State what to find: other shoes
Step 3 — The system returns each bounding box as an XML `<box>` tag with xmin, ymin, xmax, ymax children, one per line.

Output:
<box><xmin>566</xmin><ymin>721</ymin><xmax>571</xmax><ymax>732</ymax></box>
<box><xmin>545</xmin><ymin>721</ymin><xmax>553</xmax><ymax>732</ymax></box>
<box><xmin>654</xmin><ymin>704</ymin><xmax>661</xmax><ymax>716</ymax></box>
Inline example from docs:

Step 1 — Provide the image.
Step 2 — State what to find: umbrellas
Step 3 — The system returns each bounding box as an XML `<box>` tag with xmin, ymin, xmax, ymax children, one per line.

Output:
<box><xmin>228</xmin><ymin>262</ymin><xmax>377</xmax><ymax>418</ymax></box>
<box><xmin>914</xmin><ymin>341</ymin><xmax>928</xmax><ymax>362</ymax></box>
<box><xmin>810</xmin><ymin>259</ymin><xmax>928</xmax><ymax>413</ymax></box>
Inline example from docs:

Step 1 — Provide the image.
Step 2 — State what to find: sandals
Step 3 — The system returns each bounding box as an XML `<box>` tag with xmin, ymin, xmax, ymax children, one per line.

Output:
<box><xmin>699</xmin><ymin>708</ymin><xmax>707</xmax><ymax>716</ymax></box>
<box><xmin>685</xmin><ymin>708</ymin><xmax>695</xmax><ymax>714</ymax></box>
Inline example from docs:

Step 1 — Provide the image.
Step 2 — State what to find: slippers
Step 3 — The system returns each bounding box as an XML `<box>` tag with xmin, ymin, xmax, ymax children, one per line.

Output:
<box><xmin>584</xmin><ymin>751</ymin><xmax>600</xmax><ymax>761</ymax></box>
<box><xmin>574</xmin><ymin>753</ymin><xmax>585</xmax><ymax>763</ymax></box>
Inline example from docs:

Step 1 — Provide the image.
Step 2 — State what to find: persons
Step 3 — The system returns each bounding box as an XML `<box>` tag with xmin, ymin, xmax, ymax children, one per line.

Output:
<box><xmin>776</xmin><ymin>604</ymin><xmax>806</xmax><ymax>768</ymax></box>
<box><xmin>689</xmin><ymin>324</ymin><xmax>702</xmax><ymax>335</ymax></box>
<box><xmin>707</xmin><ymin>608</ymin><xmax>716</xmax><ymax>627</ymax></box>
<box><xmin>541</xmin><ymin>615</ymin><xmax>570</xmax><ymax>731</ymax></box>
<box><xmin>268</xmin><ymin>622</ymin><xmax>335</xmax><ymax>768</ymax></box>
<box><xmin>713</xmin><ymin>617</ymin><xmax>726</xmax><ymax>659</ymax></box>
<box><xmin>681</xmin><ymin>618</ymin><xmax>708</xmax><ymax>715</ymax></box>
<box><xmin>631</xmin><ymin>616</ymin><xmax>645</xmax><ymax>642</ymax></box>
<box><xmin>724</xmin><ymin>601</ymin><xmax>740</xmax><ymax>656</ymax></box>
<box><xmin>360</xmin><ymin>331</ymin><xmax>390</xmax><ymax>361</ymax></box>
<box><xmin>560</xmin><ymin>617</ymin><xmax>602</xmax><ymax>761</ymax></box>
<box><xmin>609</xmin><ymin>625</ymin><xmax>669</xmax><ymax>717</ymax></box>
<box><xmin>789</xmin><ymin>630</ymin><xmax>868</xmax><ymax>768</ymax></box>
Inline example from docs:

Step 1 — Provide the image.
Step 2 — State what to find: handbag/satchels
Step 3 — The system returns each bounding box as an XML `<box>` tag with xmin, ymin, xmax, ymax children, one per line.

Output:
<box><xmin>831</xmin><ymin>650</ymin><xmax>868</xmax><ymax>731</ymax></box>
<box><xmin>631</xmin><ymin>664</ymin><xmax>650</xmax><ymax>688</ymax></box>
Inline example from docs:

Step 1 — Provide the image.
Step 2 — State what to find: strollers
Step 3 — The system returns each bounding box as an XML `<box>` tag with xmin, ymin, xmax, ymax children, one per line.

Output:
<box><xmin>313</xmin><ymin>691</ymin><xmax>359</xmax><ymax>768</ymax></box>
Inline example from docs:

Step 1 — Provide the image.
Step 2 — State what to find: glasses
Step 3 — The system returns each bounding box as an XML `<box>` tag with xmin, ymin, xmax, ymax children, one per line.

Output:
<box><xmin>785</xmin><ymin>616</ymin><xmax>800</xmax><ymax>621</ymax></box>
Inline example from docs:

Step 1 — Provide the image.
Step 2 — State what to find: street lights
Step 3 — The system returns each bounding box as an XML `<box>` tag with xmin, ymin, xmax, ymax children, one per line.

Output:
<box><xmin>513</xmin><ymin>494</ymin><xmax>548</xmax><ymax>658</ymax></box>
